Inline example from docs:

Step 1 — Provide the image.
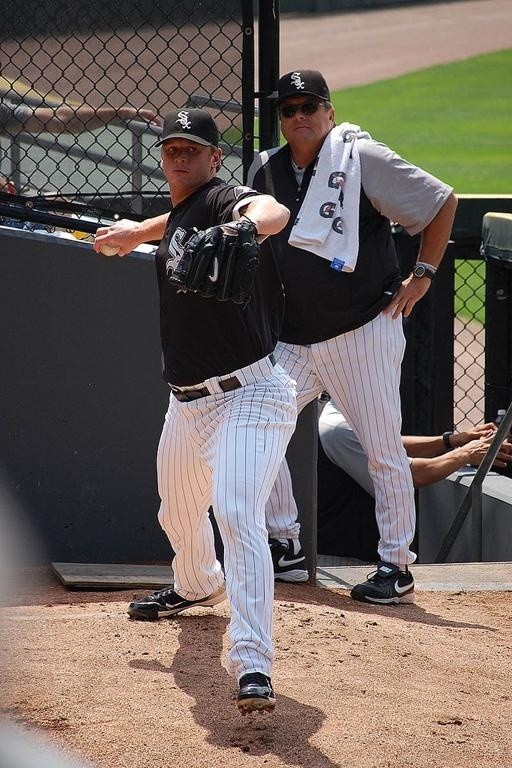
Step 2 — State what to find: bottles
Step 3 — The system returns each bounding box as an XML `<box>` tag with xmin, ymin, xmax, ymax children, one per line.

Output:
<box><xmin>495</xmin><ymin>409</ymin><xmax>507</xmax><ymax>428</ymax></box>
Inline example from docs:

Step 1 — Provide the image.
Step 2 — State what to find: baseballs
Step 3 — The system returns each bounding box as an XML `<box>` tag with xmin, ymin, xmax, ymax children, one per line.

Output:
<box><xmin>99</xmin><ymin>242</ymin><xmax>122</xmax><ymax>257</ymax></box>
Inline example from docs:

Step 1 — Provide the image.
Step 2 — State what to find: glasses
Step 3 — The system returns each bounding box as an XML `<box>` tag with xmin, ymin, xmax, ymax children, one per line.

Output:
<box><xmin>281</xmin><ymin>98</ymin><xmax>324</xmax><ymax>117</ymax></box>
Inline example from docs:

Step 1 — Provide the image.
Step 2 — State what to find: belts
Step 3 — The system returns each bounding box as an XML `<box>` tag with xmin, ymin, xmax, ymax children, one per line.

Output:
<box><xmin>172</xmin><ymin>354</ymin><xmax>276</xmax><ymax>402</ymax></box>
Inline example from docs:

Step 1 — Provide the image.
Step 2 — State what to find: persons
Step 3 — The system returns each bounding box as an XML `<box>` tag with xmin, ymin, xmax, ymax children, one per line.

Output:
<box><xmin>1</xmin><ymin>96</ymin><xmax>165</xmax><ymax>138</ymax></box>
<box><xmin>247</xmin><ymin>68</ymin><xmax>458</xmax><ymax>607</ymax></box>
<box><xmin>316</xmin><ymin>390</ymin><xmax>511</xmax><ymax>567</ymax></box>
<box><xmin>91</xmin><ymin>106</ymin><xmax>300</xmax><ymax>718</ymax></box>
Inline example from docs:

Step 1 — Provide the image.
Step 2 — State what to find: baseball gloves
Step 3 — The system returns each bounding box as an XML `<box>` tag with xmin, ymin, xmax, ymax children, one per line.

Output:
<box><xmin>169</xmin><ymin>218</ymin><xmax>262</xmax><ymax>314</ymax></box>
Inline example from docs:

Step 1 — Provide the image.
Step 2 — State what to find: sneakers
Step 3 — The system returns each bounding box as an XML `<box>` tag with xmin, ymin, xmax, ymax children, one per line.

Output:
<box><xmin>350</xmin><ymin>561</ymin><xmax>416</xmax><ymax>604</ymax></box>
<box><xmin>129</xmin><ymin>578</ymin><xmax>226</xmax><ymax>620</ymax></box>
<box><xmin>269</xmin><ymin>537</ymin><xmax>310</xmax><ymax>583</ymax></box>
<box><xmin>237</xmin><ymin>672</ymin><xmax>276</xmax><ymax>712</ymax></box>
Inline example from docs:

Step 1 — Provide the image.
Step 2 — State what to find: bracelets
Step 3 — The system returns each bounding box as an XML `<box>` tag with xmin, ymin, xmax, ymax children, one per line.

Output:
<box><xmin>416</xmin><ymin>260</ymin><xmax>437</xmax><ymax>275</ymax></box>
<box><xmin>441</xmin><ymin>430</ymin><xmax>454</xmax><ymax>451</ymax></box>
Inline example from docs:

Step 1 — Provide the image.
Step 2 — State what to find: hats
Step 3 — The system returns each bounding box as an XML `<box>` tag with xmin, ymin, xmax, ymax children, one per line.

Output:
<box><xmin>273</xmin><ymin>70</ymin><xmax>329</xmax><ymax>105</ymax></box>
<box><xmin>154</xmin><ymin>108</ymin><xmax>219</xmax><ymax>149</ymax></box>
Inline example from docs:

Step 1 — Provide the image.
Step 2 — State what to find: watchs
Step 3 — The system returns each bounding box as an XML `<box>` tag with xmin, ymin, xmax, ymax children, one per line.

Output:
<box><xmin>409</xmin><ymin>264</ymin><xmax>436</xmax><ymax>282</ymax></box>
